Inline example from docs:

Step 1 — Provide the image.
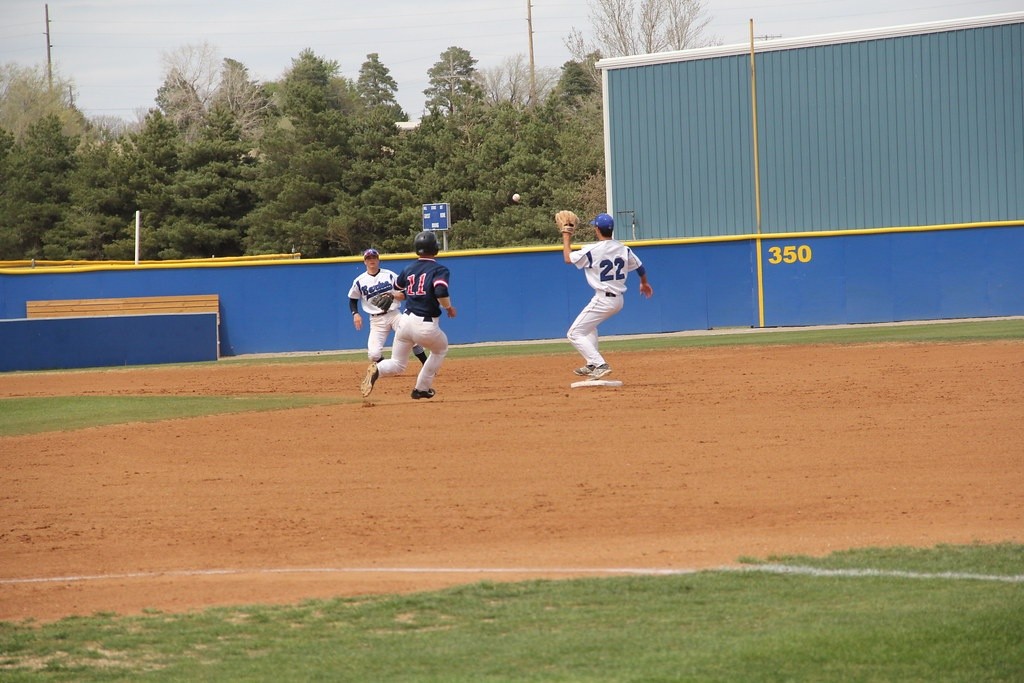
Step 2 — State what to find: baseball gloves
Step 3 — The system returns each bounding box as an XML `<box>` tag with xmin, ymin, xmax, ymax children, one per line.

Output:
<box><xmin>371</xmin><ymin>292</ymin><xmax>395</xmax><ymax>313</ymax></box>
<box><xmin>555</xmin><ymin>209</ymin><xmax>581</xmax><ymax>235</ymax></box>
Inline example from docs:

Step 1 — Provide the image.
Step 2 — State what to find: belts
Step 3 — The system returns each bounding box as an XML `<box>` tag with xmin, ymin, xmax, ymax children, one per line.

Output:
<box><xmin>369</xmin><ymin>311</ymin><xmax>388</xmax><ymax>317</ymax></box>
<box><xmin>403</xmin><ymin>309</ymin><xmax>433</xmax><ymax>322</ymax></box>
<box><xmin>605</xmin><ymin>291</ymin><xmax>624</xmax><ymax>297</ymax></box>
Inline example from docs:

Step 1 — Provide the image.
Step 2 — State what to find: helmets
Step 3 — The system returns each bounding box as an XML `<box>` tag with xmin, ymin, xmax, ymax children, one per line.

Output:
<box><xmin>413</xmin><ymin>231</ymin><xmax>440</xmax><ymax>256</ymax></box>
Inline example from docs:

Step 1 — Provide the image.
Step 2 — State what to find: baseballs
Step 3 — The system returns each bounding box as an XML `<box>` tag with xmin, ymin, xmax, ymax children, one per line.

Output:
<box><xmin>512</xmin><ymin>193</ymin><xmax>520</xmax><ymax>201</ymax></box>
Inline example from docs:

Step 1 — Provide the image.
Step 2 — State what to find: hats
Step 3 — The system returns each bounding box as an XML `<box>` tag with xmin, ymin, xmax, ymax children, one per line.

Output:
<box><xmin>590</xmin><ymin>213</ymin><xmax>615</xmax><ymax>230</ymax></box>
<box><xmin>364</xmin><ymin>248</ymin><xmax>380</xmax><ymax>259</ymax></box>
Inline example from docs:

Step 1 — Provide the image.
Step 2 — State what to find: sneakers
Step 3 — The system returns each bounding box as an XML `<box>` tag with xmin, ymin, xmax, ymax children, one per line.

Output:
<box><xmin>573</xmin><ymin>365</ymin><xmax>596</xmax><ymax>375</ymax></box>
<box><xmin>590</xmin><ymin>364</ymin><xmax>612</xmax><ymax>379</ymax></box>
<box><xmin>359</xmin><ymin>363</ymin><xmax>380</xmax><ymax>397</ymax></box>
<box><xmin>410</xmin><ymin>388</ymin><xmax>436</xmax><ymax>399</ymax></box>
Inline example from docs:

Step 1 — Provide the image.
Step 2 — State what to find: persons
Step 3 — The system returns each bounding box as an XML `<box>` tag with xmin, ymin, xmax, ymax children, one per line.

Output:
<box><xmin>361</xmin><ymin>231</ymin><xmax>456</xmax><ymax>399</ymax></box>
<box><xmin>348</xmin><ymin>249</ymin><xmax>428</xmax><ymax>364</ymax></box>
<box><xmin>556</xmin><ymin>210</ymin><xmax>653</xmax><ymax>381</ymax></box>
